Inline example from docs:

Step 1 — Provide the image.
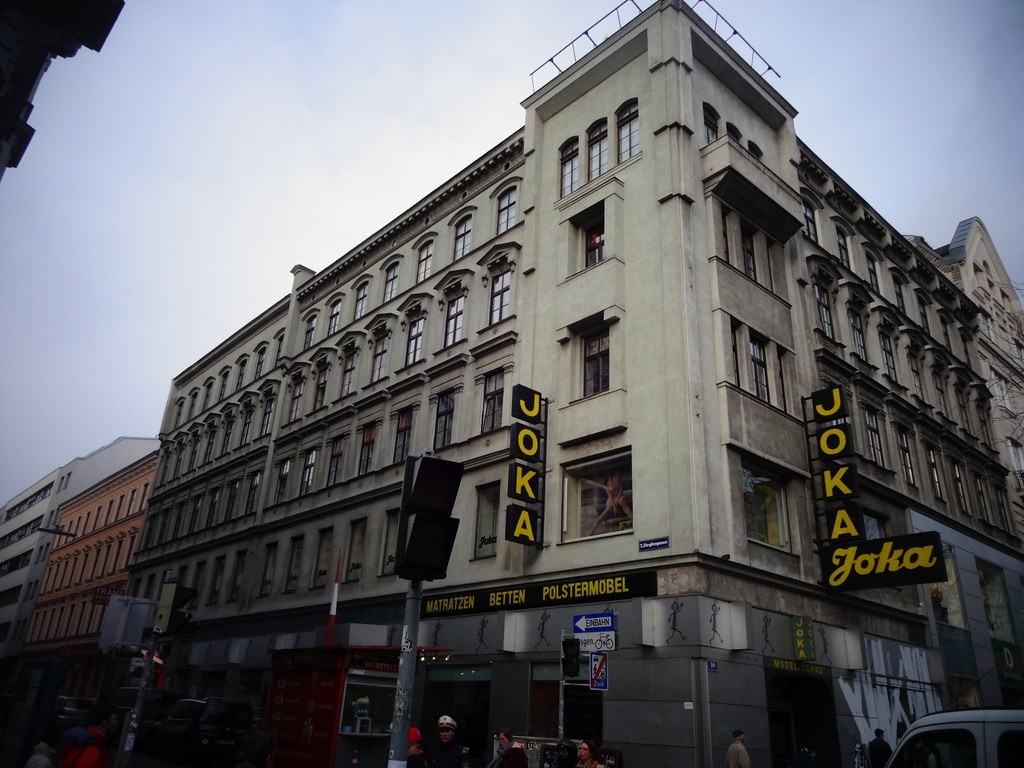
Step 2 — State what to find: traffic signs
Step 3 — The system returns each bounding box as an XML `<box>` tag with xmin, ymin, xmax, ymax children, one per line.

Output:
<box><xmin>572</xmin><ymin>611</ymin><xmax>616</xmax><ymax>632</ymax></box>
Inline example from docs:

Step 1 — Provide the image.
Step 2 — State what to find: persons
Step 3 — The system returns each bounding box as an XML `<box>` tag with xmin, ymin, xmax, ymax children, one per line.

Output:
<box><xmin>426</xmin><ymin>715</ymin><xmax>462</xmax><ymax>768</ymax></box>
<box><xmin>725</xmin><ymin>728</ymin><xmax>752</xmax><ymax>768</ymax></box>
<box><xmin>589</xmin><ymin>469</ymin><xmax>633</xmax><ymax>537</ymax></box>
<box><xmin>868</xmin><ymin>728</ymin><xmax>893</xmax><ymax>768</ymax></box>
<box><xmin>487</xmin><ymin>727</ymin><xmax>529</xmax><ymax>768</ymax></box>
<box><xmin>575</xmin><ymin>740</ymin><xmax>605</xmax><ymax>768</ymax></box>
<box><xmin>788</xmin><ymin>743</ymin><xmax>821</xmax><ymax>768</ymax></box>
<box><xmin>406</xmin><ymin>727</ymin><xmax>426</xmax><ymax>767</ymax></box>
<box><xmin>556</xmin><ymin>739</ymin><xmax>580</xmax><ymax>768</ymax></box>
<box><xmin>24</xmin><ymin>701</ymin><xmax>121</xmax><ymax>768</ymax></box>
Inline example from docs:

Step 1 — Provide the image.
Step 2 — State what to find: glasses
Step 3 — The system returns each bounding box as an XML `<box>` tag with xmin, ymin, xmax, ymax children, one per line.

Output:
<box><xmin>579</xmin><ymin>747</ymin><xmax>590</xmax><ymax>751</ymax></box>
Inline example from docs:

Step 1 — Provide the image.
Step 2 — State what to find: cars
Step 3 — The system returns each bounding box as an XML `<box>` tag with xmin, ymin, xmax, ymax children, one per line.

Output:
<box><xmin>53</xmin><ymin>694</ymin><xmax>94</xmax><ymax>726</ymax></box>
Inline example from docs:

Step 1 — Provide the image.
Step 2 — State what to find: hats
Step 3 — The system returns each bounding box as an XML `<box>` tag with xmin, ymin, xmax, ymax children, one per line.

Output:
<box><xmin>732</xmin><ymin>728</ymin><xmax>746</xmax><ymax>738</ymax></box>
<box><xmin>437</xmin><ymin>715</ymin><xmax>457</xmax><ymax>731</ymax></box>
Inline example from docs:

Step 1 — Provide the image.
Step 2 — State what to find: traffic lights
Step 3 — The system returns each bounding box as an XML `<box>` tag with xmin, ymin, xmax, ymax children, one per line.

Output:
<box><xmin>397</xmin><ymin>451</ymin><xmax>466</xmax><ymax>588</ymax></box>
<box><xmin>560</xmin><ymin>638</ymin><xmax>582</xmax><ymax>678</ymax></box>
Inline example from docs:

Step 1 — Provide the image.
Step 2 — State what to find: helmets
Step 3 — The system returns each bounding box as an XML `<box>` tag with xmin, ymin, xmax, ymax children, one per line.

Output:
<box><xmin>556</xmin><ymin>738</ymin><xmax>578</xmax><ymax>755</ymax></box>
<box><xmin>407</xmin><ymin>726</ymin><xmax>422</xmax><ymax>746</ymax></box>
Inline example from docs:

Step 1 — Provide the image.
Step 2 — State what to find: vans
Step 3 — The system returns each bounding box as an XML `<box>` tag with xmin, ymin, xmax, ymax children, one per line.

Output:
<box><xmin>883</xmin><ymin>707</ymin><xmax>1024</xmax><ymax>767</ymax></box>
<box><xmin>146</xmin><ymin>696</ymin><xmax>256</xmax><ymax>763</ymax></box>
